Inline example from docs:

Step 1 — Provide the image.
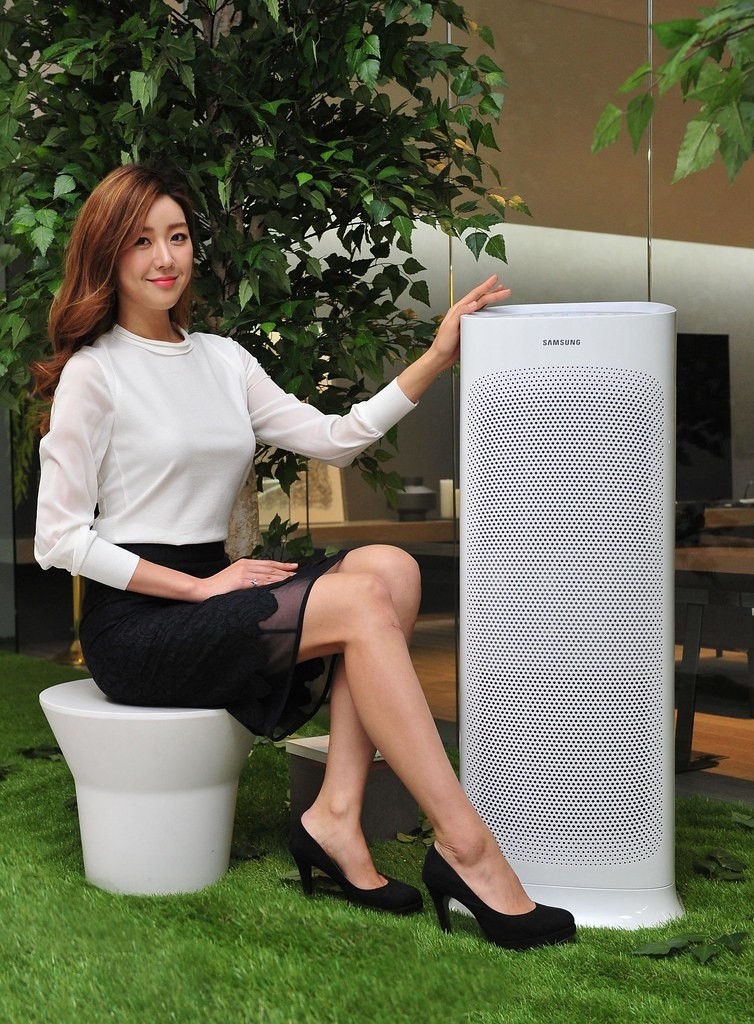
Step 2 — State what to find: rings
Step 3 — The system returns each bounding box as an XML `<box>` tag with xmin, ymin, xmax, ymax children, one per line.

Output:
<box><xmin>251</xmin><ymin>579</ymin><xmax>258</xmax><ymax>588</ymax></box>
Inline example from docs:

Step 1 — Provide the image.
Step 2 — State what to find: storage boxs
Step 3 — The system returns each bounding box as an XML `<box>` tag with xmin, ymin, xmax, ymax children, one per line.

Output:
<box><xmin>283</xmin><ymin>729</ymin><xmax>432</xmax><ymax>840</ymax></box>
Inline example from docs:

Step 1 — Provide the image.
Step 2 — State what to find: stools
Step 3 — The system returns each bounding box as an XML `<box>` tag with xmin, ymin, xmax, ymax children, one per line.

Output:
<box><xmin>38</xmin><ymin>674</ymin><xmax>256</xmax><ymax>895</ymax></box>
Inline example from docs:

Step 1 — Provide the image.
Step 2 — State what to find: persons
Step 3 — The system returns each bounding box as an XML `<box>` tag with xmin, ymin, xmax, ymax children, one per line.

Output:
<box><xmin>32</xmin><ymin>163</ymin><xmax>579</xmax><ymax>952</ymax></box>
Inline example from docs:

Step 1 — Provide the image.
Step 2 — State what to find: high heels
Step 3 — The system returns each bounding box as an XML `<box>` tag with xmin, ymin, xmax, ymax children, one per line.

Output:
<box><xmin>288</xmin><ymin>817</ymin><xmax>423</xmax><ymax>912</ymax></box>
<box><xmin>420</xmin><ymin>837</ymin><xmax>576</xmax><ymax>950</ymax></box>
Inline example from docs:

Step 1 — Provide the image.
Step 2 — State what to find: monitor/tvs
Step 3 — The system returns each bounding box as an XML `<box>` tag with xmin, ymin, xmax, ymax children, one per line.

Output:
<box><xmin>675</xmin><ymin>333</ymin><xmax>734</xmax><ymax>503</ymax></box>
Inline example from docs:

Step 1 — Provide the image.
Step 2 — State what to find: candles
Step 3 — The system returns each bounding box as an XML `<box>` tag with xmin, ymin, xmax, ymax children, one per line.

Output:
<box><xmin>453</xmin><ymin>489</ymin><xmax>460</xmax><ymax>517</ymax></box>
<box><xmin>440</xmin><ymin>478</ymin><xmax>453</xmax><ymax>518</ymax></box>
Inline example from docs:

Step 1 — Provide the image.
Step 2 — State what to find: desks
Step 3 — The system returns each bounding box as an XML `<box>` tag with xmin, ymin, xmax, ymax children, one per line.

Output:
<box><xmin>261</xmin><ymin>517</ymin><xmax>458</xmax><ymax>546</ymax></box>
<box><xmin>675</xmin><ymin>546</ymin><xmax>754</xmax><ymax>774</ymax></box>
<box><xmin>699</xmin><ymin>507</ymin><xmax>753</xmax><ymax>545</ymax></box>
